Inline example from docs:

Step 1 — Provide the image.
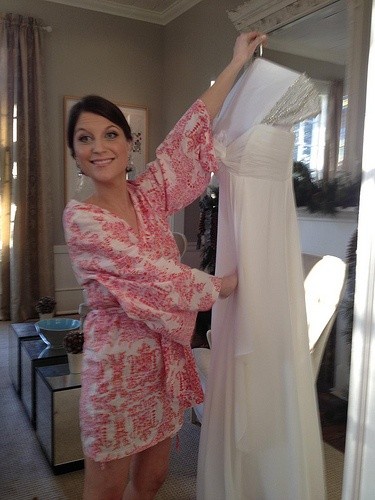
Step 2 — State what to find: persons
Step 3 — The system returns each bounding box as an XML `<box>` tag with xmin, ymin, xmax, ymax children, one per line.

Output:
<box><xmin>63</xmin><ymin>31</ymin><xmax>267</xmax><ymax>500</ymax></box>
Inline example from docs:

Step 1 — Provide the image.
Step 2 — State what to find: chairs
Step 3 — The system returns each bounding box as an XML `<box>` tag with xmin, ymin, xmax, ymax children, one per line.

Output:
<box><xmin>190</xmin><ymin>268</ymin><xmax>349</xmax><ymax>426</ymax></box>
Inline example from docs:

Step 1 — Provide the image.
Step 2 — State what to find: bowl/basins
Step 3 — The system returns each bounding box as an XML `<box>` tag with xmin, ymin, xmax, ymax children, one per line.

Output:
<box><xmin>34</xmin><ymin>318</ymin><xmax>81</xmax><ymax>350</ymax></box>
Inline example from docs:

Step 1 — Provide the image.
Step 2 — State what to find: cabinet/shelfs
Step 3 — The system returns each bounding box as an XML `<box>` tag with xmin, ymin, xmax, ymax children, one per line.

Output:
<box><xmin>52</xmin><ymin>245</ymin><xmax>85</xmax><ymax>315</ymax></box>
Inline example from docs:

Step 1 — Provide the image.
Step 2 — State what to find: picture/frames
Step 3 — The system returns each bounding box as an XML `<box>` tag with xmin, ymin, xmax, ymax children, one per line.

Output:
<box><xmin>63</xmin><ymin>95</ymin><xmax>149</xmax><ymax>208</ymax></box>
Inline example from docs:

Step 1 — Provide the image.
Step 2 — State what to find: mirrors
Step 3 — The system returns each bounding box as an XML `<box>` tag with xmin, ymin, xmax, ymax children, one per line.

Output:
<box><xmin>226</xmin><ymin>1</ymin><xmax>375</xmax><ymax>209</ymax></box>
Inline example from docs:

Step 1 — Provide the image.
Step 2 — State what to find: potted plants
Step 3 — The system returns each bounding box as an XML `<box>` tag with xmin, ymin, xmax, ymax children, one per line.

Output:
<box><xmin>34</xmin><ymin>298</ymin><xmax>58</xmax><ymax>320</ymax></box>
<box><xmin>64</xmin><ymin>331</ymin><xmax>86</xmax><ymax>374</ymax></box>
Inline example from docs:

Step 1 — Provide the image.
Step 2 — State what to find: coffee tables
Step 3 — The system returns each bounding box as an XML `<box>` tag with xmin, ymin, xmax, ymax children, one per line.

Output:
<box><xmin>9</xmin><ymin>322</ymin><xmax>85</xmax><ymax>475</ymax></box>
